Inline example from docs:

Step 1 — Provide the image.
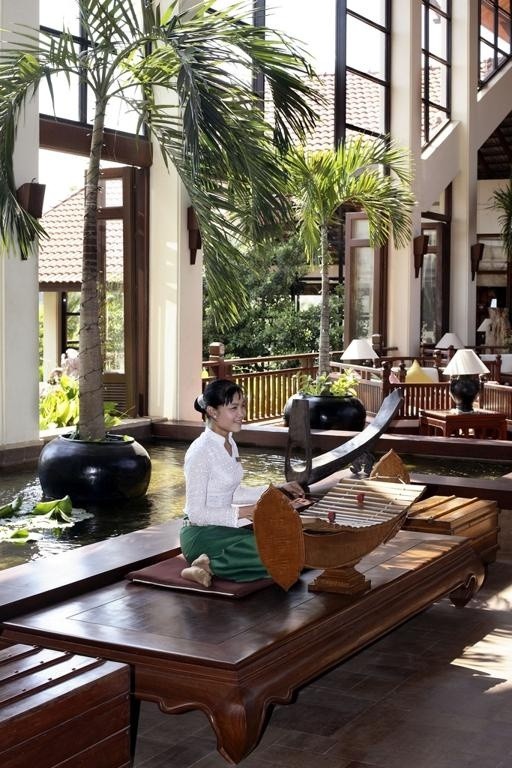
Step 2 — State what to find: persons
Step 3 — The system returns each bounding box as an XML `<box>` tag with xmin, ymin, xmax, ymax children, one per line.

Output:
<box><xmin>484</xmin><ymin>306</ymin><xmax>511</xmax><ymax>354</ymax></box>
<box><xmin>179</xmin><ymin>378</ymin><xmax>308</xmax><ymax>587</ymax></box>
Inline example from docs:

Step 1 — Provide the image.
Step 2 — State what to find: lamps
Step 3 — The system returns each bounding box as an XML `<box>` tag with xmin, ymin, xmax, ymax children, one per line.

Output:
<box><xmin>435</xmin><ymin>318</ymin><xmax>497</xmax><ymax>412</ymax></box>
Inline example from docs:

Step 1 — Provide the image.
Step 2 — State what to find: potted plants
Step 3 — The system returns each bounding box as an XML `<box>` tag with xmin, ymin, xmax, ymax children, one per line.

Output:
<box><xmin>230</xmin><ymin>134</ymin><xmax>418</xmax><ymax>431</ymax></box>
<box><xmin>0</xmin><ymin>0</ymin><xmax>327</xmax><ymax>510</ymax></box>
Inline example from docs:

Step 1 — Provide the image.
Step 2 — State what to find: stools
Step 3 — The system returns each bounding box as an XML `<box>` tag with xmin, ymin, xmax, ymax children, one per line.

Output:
<box><xmin>398</xmin><ymin>493</ymin><xmax>502</xmax><ymax>575</ymax></box>
<box><xmin>0</xmin><ymin>643</ymin><xmax>135</xmax><ymax>768</ymax></box>
